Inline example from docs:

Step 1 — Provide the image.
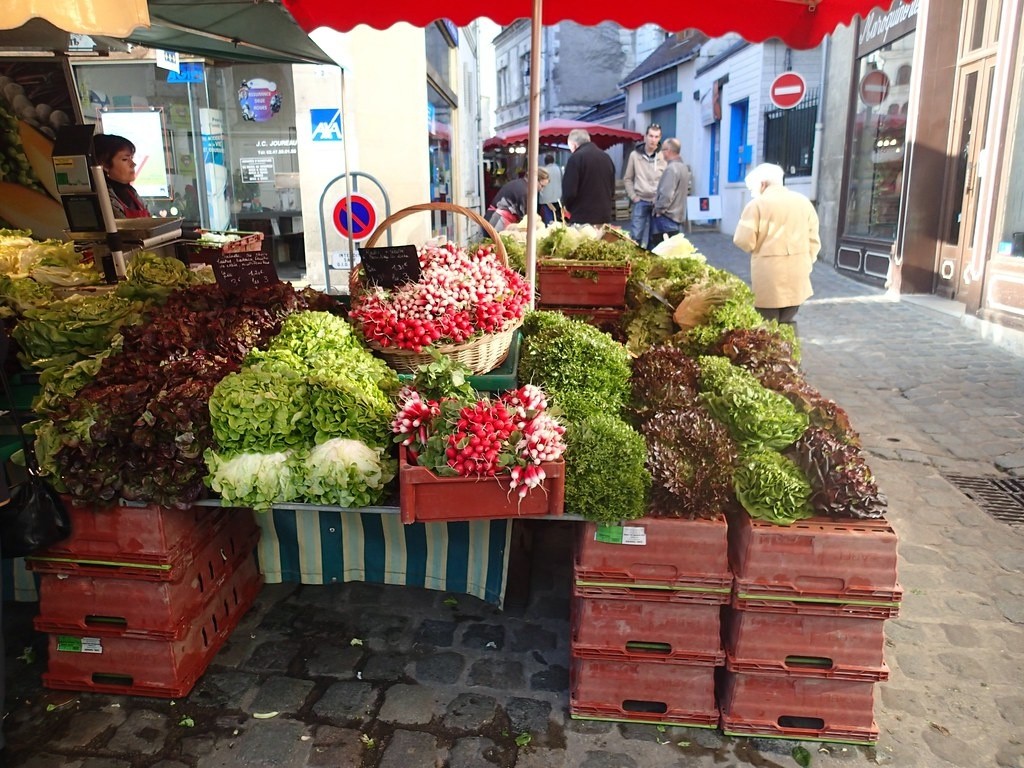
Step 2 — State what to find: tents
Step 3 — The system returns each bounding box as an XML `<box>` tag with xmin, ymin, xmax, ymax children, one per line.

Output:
<box><xmin>0</xmin><ymin>0</ymin><xmax>354</xmax><ymax>277</ymax></box>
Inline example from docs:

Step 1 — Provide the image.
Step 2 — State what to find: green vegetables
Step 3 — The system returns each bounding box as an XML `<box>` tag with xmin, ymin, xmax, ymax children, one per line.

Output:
<box><xmin>475</xmin><ymin>228</ymin><xmax>801</xmax><ymax>363</ymax></box>
<box><xmin>518</xmin><ymin>311</ymin><xmax>891</xmax><ymax>526</ymax></box>
<box><xmin>0</xmin><ymin>226</ymin><xmax>409</xmax><ymax>515</ymax></box>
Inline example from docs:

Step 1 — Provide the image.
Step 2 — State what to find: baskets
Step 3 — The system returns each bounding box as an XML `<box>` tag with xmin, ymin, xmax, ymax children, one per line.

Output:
<box><xmin>350</xmin><ymin>202</ymin><xmax>525</xmax><ymax>376</ymax></box>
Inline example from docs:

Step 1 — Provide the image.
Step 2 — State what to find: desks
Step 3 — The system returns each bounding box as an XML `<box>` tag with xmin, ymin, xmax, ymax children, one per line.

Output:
<box><xmin>240</xmin><ymin>216</ymin><xmax>293</xmax><ymax>258</ymax></box>
<box><xmin>118</xmin><ymin>490</ymin><xmax>627</xmax><ymax>524</ymax></box>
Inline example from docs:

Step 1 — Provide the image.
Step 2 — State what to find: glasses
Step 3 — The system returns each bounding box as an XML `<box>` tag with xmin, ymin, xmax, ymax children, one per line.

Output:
<box><xmin>538</xmin><ymin>180</ymin><xmax>544</xmax><ymax>188</ymax></box>
<box><xmin>651</xmin><ymin>123</ymin><xmax>661</xmax><ymax>128</ymax></box>
<box><xmin>661</xmin><ymin>148</ymin><xmax>669</xmax><ymax>152</ymax></box>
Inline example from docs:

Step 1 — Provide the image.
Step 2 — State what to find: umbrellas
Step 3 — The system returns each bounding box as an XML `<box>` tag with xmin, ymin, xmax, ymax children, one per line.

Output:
<box><xmin>483</xmin><ymin>117</ymin><xmax>643</xmax><ymax>165</ymax></box>
<box><xmin>283</xmin><ymin>0</ymin><xmax>909</xmax><ymax>316</ymax></box>
<box><xmin>428</xmin><ymin>121</ymin><xmax>451</xmax><ymax>153</ymax></box>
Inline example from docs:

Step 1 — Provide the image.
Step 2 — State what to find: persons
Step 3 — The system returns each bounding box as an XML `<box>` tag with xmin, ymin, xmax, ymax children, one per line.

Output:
<box><xmin>651</xmin><ymin>136</ymin><xmax>690</xmax><ymax>231</ymax></box>
<box><xmin>90</xmin><ymin>134</ymin><xmax>150</xmax><ymax>219</ymax></box>
<box><xmin>561</xmin><ymin>128</ymin><xmax>618</xmax><ymax>225</ymax></box>
<box><xmin>732</xmin><ymin>162</ymin><xmax>821</xmax><ymax>344</ymax></box>
<box><xmin>540</xmin><ymin>154</ymin><xmax>562</xmax><ymax>225</ymax></box>
<box><xmin>624</xmin><ymin>126</ymin><xmax>668</xmax><ymax>249</ymax></box>
<box><xmin>478</xmin><ymin>167</ymin><xmax>552</xmax><ymax>239</ymax></box>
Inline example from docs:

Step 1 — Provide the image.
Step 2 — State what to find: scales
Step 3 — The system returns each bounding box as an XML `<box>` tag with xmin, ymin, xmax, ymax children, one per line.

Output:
<box><xmin>50</xmin><ymin>123</ymin><xmax>187</xmax><ymax>285</ymax></box>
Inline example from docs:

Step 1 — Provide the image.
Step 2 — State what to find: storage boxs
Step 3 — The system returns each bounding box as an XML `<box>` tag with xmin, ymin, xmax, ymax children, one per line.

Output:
<box><xmin>569</xmin><ymin>515</ymin><xmax>905</xmax><ymax>747</ymax></box>
<box><xmin>180</xmin><ymin>229</ymin><xmax>263</xmax><ymax>268</ymax></box>
<box><xmin>536</xmin><ymin>256</ymin><xmax>631</xmax><ymax>341</ymax></box>
<box><xmin>27</xmin><ymin>500</ymin><xmax>259</xmax><ymax>699</ymax></box>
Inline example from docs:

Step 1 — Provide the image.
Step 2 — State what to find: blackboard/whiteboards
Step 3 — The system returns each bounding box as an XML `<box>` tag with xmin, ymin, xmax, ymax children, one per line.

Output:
<box><xmin>684</xmin><ymin>196</ymin><xmax>722</xmax><ymax>233</ymax></box>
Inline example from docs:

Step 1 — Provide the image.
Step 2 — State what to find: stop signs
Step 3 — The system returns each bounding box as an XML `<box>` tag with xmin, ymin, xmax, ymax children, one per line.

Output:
<box><xmin>769</xmin><ymin>71</ymin><xmax>807</xmax><ymax>109</ymax></box>
<box><xmin>858</xmin><ymin>69</ymin><xmax>891</xmax><ymax>108</ymax></box>
